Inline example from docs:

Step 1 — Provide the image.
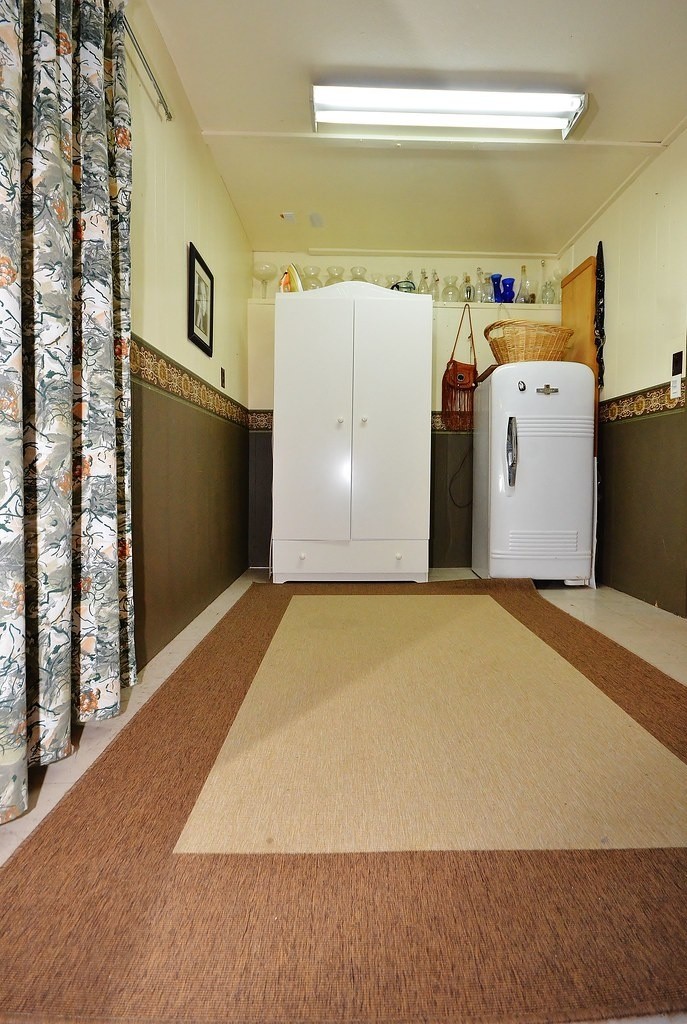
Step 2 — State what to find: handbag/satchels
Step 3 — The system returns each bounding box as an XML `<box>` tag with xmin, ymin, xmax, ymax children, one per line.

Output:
<box><xmin>442</xmin><ymin>304</ymin><xmax>479</xmax><ymax>432</ymax></box>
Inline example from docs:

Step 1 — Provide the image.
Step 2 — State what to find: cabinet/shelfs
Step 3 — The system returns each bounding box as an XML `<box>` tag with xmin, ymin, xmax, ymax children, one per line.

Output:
<box><xmin>272</xmin><ymin>281</ymin><xmax>432</xmax><ymax>584</ymax></box>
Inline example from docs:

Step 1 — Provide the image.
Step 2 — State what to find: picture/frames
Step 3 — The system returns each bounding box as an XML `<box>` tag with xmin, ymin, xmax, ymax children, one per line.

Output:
<box><xmin>188</xmin><ymin>242</ymin><xmax>214</xmax><ymax>358</ymax></box>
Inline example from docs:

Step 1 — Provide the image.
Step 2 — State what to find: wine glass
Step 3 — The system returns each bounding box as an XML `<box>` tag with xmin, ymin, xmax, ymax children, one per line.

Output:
<box><xmin>249</xmin><ymin>261</ymin><xmax>279</xmax><ymax>299</ymax></box>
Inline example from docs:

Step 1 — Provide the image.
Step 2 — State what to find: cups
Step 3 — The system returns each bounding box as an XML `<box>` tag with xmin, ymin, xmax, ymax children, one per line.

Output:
<box><xmin>527</xmin><ymin>280</ymin><xmax>539</xmax><ymax>303</ymax></box>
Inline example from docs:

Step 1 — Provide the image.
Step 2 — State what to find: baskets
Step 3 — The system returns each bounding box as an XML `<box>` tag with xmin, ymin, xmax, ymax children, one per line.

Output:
<box><xmin>484</xmin><ymin>319</ymin><xmax>574</xmax><ymax>365</ymax></box>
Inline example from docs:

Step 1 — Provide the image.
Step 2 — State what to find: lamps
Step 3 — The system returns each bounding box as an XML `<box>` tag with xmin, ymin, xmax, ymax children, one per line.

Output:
<box><xmin>311</xmin><ymin>83</ymin><xmax>590</xmax><ymax>139</ymax></box>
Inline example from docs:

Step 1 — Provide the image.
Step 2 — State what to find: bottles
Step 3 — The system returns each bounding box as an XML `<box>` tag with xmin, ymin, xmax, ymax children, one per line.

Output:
<box><xmin>429</xmin><ymin>269</ymin><xmax>439</xmax><ymax>302</ymax></box>
<box><xmin>349</xmin><ymin>266</ymin><xmax>369</xmax><ymax>283</ymax></box>
<box><xmin>474</xmin><ymin>266</ymin><xmax>485</xmax><ymax>303</ymax></box>
<box><xmin>441</xmin><ymin>276</ymin><xmax>460</xmax><ymax>302</ymax></box>
<box><xmin>482</xmin><ymin>277</ymin><xmax>494</xmax><ymax>302</ymax></box>
<box><xmin>371</xmin><ymin>273</ymin><xmax>400</xmax><ymax>291</ymax></box>
<box><xmin>541</xmin><ymin>281</ymin><xmax>555</xmax><ymax>304</ymax></box>
<box><xmin>300</xmin><ymin>264</ymin><xmax>323</xmax><ymax>291</ymax></box>
<box><xmin>459</xmin><ymin>276</ymin><xmax>476</xmax><ymax>302</ymax></box>
<box><xmin>514</xmin><ymin>264</ymin><xmax>530</xmax><ymax>303</ymax></box>
<box><xmin>324</xmin><ymin>266</ymin><xmax>345</xmax><ymax>287</ymax></box>
<box><xmin>418</xmin><ymin>267</ymin><xmax>428</xmax><ymax>294</ymax></box>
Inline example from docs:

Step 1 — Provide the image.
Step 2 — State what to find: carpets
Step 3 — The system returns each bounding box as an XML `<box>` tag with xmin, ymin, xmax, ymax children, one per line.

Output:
<box><xmin>0</xmin><ymin>578</ymin><xmax>687</xmax><ymax>1023</ymax></box>
